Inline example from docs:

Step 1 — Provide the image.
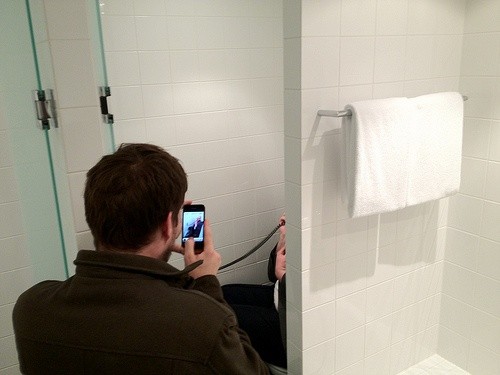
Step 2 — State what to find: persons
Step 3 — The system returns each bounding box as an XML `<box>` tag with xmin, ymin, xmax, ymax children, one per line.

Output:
<box><xmin>12</xmin><ymin>142</ymin><xmax>273</xmax><ymax>375</ymax></box>
<box><xmin>184</xmin><ymin>216</ymin><xmax>204</xmax><ymax>238</ymax></box>
<box><xmin>221</xmin><ymin>216</ymin><xmax>287</xmax><ymax>369</ymax></box>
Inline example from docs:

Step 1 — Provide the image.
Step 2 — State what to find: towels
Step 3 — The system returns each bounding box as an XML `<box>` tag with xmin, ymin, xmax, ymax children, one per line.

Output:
<box><xmin>340</xmin><ymin>91</ymin><xmax>464</xmax><ymax>219</ymax></box>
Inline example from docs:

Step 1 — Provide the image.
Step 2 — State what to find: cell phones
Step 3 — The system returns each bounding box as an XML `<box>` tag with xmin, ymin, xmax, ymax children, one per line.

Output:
<box><xmin>181</xmin><ymin>204</ymin><xmax>206</xmax><ymax>251</ymax></box>
